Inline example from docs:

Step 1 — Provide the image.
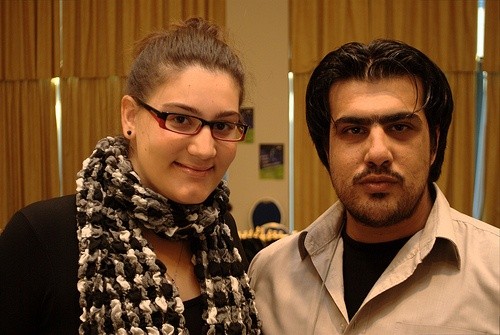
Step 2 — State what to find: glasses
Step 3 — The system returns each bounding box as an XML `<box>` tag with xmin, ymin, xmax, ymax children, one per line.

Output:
<box><xmin>133</xmin><ymin>97</ymin><xmax>248</xmax><ymax>142</ymax></box>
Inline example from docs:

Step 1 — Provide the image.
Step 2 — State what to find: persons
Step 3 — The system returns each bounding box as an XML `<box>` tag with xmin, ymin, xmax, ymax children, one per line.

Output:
<box><xmin>245</xmin><ymin>39</ymin><xmax>500</xmax><ymax>335</ymax></box>
<box><xmin>0</xmin><ymin>20</ymin><xmax>265</xmax><ymax>334</ymax></box>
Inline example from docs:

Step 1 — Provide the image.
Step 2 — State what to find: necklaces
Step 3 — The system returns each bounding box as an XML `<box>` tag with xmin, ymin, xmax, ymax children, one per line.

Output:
<box><xmin>151</xmin><ymin>239</ymin><xmax>185</xmax><ymax>285</ymax></box>
